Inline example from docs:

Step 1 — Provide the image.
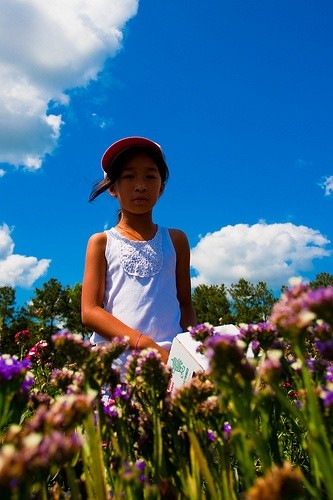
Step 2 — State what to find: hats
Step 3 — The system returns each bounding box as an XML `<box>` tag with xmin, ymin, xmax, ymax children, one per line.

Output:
<box><xmin>101</xmin><ymin>136</ymin><xmax>163</xmax><ymax>179</ymax></box>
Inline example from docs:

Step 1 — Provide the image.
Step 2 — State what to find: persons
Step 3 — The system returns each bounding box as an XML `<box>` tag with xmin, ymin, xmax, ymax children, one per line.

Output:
<box><xmin>78</xmin><ymin>136</ymin><xmax>196</xmax><ymax>365</ymax></box>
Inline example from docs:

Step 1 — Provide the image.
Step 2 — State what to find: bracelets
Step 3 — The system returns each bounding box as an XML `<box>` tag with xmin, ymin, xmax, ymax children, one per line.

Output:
<box><xmin>135</xmin><ymin>333</ymin><xmax>143</xmax><ymax>350</ymax></box>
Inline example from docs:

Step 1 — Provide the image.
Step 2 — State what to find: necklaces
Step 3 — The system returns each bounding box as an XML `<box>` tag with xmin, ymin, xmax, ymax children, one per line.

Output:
<box><xmin>117</xmin><ymin>223</ymin><xmax>158</xmax><ymax>241</ymax></box>
